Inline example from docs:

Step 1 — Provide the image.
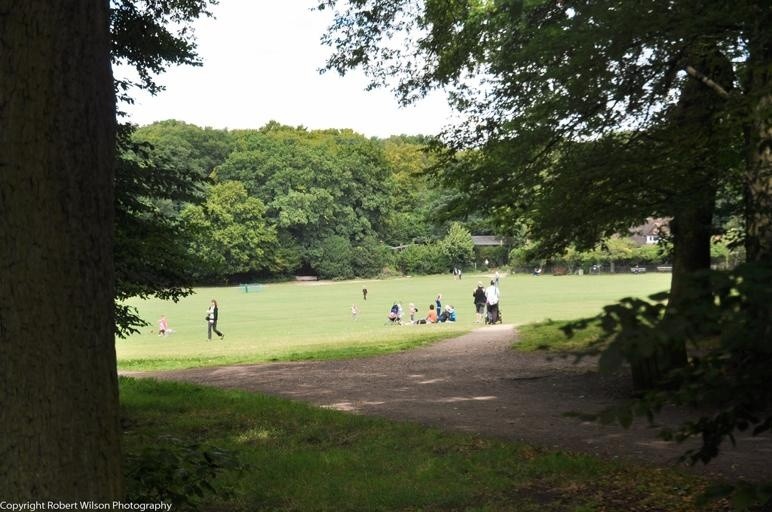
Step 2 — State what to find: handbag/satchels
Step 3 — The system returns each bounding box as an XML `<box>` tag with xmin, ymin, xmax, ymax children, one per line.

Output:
<box><xmin>206</xmin><ymin>317</ymin><xmax>210</xmax><ymax>321</ymax></box>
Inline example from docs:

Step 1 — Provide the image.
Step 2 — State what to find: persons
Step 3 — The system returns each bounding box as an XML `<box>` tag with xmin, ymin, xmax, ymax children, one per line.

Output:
<box><xmin>458</xmin><ymin>269</ymin><xmax>462</xmax><ymax>280</ymax></box>
<box><xmin>635</xmin><ymin>265</ymin><xmax>640</xmax><ymax>274</ymax></box>
<box><xmin>579</xmin><ymin>263</ymin><xmax>585</xmax><ymax>275</ymax></box>
<box><xmin>389</xmin><ymin>293</ymin><xmax>456</xmax><ymax>324</ymax></box>
<box><xmin>473</xmin><ymin>271</ymin><xmax>500</xmax><ymax>325</ymax></box>
<box><xmin>453</xmin><ymin>266</ymin><xmax>457</xmax><ymax>280</ymax></box>
<box><xmin>158</xmin><ymin>314</ymin><xmax>168</xmax><ymax>336</ymax></box>
<box><xmin>206</xmin><ymin>299</ymin><xmax>224</xmax><ymax>341</ymax></box>
<box><xmin>484</xmin><ymin>258</ymin><xmax>490</xmax><ymax>266</ymax></box>
<box><xmin>363</xmin><ymin>287</ymin><xmax>368</xmax><ymax>301</ymax></box>
<box><xmin>352</xmin><ymin>303</ymin><xmax>356</xmax><ymax>320</ymax></box>
<box><xmin>591</xmin><ymin>264</ymin><xmax>598</xmax><ymax>274</ymax></box>
<box><xmin>534</xmin><ymin>265</ymin><xmax>541</xmax><ymax>276</ymax></box>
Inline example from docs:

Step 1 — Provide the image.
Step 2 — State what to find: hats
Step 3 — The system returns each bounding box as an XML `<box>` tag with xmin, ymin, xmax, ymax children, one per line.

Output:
<box><xmin>479</xmin><ymin>282</ymin><xmax>483</xmax><ymax>287</ymax></box>
<box><xmin>491</xmin><ymin>280</ymin><xmax>495</xmax><ymax>284</ymax></box>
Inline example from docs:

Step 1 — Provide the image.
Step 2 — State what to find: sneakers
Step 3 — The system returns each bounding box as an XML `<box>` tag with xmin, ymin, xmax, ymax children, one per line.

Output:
<box><xmin>207</xmin><ymin>338</ymin><xmax>210</xmax><ymax>341</ymax></box>
<box><xmin>488</xmin><ymin>318</ymin><xmax>496</xmax><ymax>324</ymax></box>
<box><xmin>220</xmin><ymin>334</ymin><xmax>224</xmax><ymax>340</ymax></box>
